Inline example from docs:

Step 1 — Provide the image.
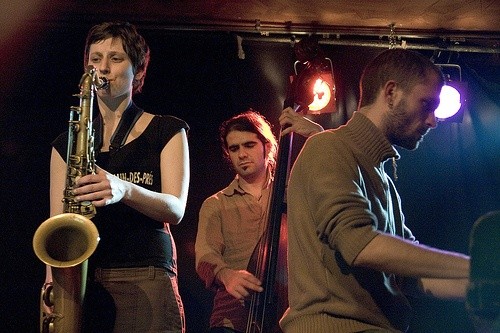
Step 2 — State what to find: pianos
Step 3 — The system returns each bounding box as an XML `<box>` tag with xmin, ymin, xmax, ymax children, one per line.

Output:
<box><xmin>463</xmin><ymin>212</ymin><xmax>500</xmax><ymax>333</ymax></box>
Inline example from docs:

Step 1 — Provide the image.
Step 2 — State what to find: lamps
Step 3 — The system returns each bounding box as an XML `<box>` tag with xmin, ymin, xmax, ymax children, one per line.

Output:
<box><xmin>431</xmin><ymin>62</ymin><xmax>465</xmax><ymax>123</ymax></box>
<box><xmin>292</xmin><ymin>38</ymin><xmax>337</xmax><ymax>116</ymax></box>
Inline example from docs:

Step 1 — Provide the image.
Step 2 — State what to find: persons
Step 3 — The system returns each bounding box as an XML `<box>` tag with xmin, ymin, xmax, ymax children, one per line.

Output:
<box><xmin>41</xmin><ymin>21</ymin><xmax>190</xmax><ymax>333</ymax></box>
<box><xmin>278</xmin><ymin>47</ymin><xmax>471</xmax><ymax>333</ymax></box>
<box><xmin>193</xmin><ymin>107</ymin><xmax>324</xmax><ymax>333</ymax></box>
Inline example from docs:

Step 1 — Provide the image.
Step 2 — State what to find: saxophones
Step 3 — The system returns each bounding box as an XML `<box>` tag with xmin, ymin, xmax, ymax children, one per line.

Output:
<box><xmin>32</xmin><ymin>64</ymin><xmax>101</xmax><ymax>333</ymax></box>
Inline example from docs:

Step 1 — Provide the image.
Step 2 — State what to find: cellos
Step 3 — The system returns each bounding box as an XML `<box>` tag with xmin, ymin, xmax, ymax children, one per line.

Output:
<box><xmin>240</xmin><ymin>32</ymin><xmax>326</xmax><ymax>333</ymax></box>
